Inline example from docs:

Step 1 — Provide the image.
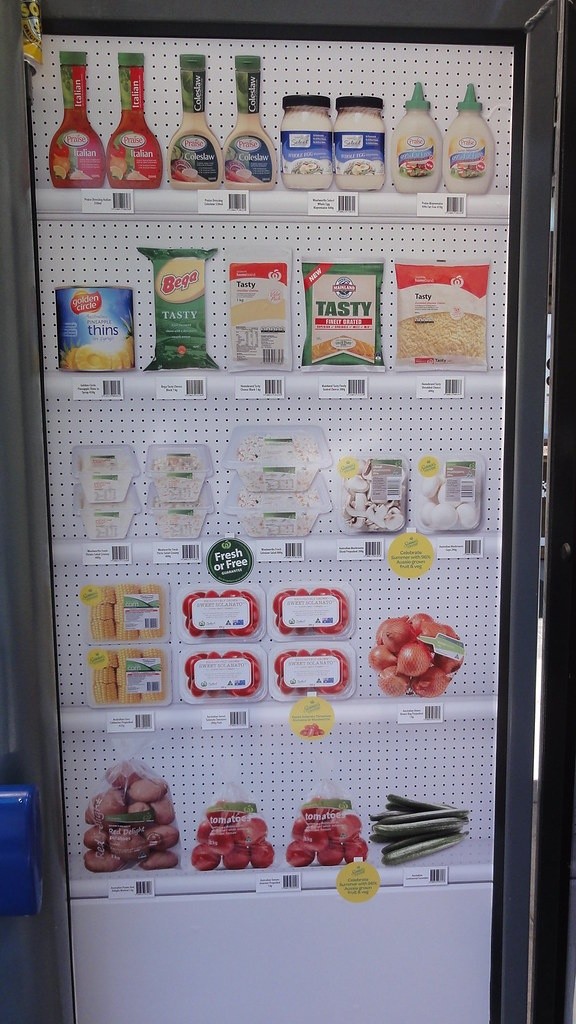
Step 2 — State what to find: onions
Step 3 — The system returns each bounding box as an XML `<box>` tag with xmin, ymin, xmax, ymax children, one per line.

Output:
<box><xmin>368</xmin><ymin>610</ymin><xmax>464</xmax><ymax>699</ymax></box>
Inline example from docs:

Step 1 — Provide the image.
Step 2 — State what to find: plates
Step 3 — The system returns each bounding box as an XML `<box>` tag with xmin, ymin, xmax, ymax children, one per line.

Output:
<box><xmin>336</xmin><ymin>450</ymin><xmax>409</xmax><ymax>536</ymax></box>
<box><xmin>269</xmin><ymin>641</ymin><xmax>356</xmax><ymax>702</ymax></box>
<box><xmin>413</xmin><ymin>452</ymin><xmax>486</xmax><ymax>535</ymax></box>
<box><xmin>80</xmin><ymin>581</ymin><xmax>170</xmax><ymax>644</ymax></box>
<box><xmin>178</xmin><ymin>644</ymin><xmax>268</xmax><ymax>705</ymax></box>
<box><xmin>85</xmin><ymin>646</ymin><xmax>172</xmax><ymax>708</ymax></box>
<box><xmin>174</xmin><ymin>583</ymin><xmax>267</xmax><ymax>645</ymax></box>
<box><xmin>266</xmin><ymin>581</ymin><xmax>356</xmax><ymax>642</ymax></box>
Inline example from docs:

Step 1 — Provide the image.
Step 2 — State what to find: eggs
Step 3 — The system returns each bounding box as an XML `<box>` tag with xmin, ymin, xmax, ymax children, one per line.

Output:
<box><xmin>418</xmin><ymin>468</ymin><xmax>479</xmax><ymax>530</ymax></box>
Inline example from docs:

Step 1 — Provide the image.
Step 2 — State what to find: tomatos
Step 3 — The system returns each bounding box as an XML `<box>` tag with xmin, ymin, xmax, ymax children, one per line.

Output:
<box><xmin>284</xmin><ymin>797</ymin><xmax>368</xmax><ymax>867</ymax></box>
<box><xmin>192</xmin><ymin>801</ymin><xmax>275</xmax><ymax>870</ymax></box>
<box><xmin>182</xmin><ymin>589</ymin><xmax>261</xmax><ymax>696</ymax></box>
<box><xmin>273</xmin><ymin>588</ymin><xmax>350</xmax><ymax>695</ymax></box>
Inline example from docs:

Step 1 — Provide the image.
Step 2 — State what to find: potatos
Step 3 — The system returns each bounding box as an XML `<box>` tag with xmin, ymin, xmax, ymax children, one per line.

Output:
<box><xmin>82</xmin><ymin>762</ymin><xmax>179</xmax><ymax>872</ymax></box>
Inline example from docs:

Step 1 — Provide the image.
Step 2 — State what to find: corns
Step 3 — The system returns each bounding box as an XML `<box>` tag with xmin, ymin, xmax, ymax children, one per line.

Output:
<box><xmin>88</xmin><ymin>583</ymin><xmax>165</xmax><ymax>703</ymax></box>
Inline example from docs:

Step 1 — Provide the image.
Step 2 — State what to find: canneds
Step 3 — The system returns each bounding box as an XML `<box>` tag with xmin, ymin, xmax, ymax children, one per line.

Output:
<box><xmin>56</xmin><ymin>285</ymin><xmax>135</xmax><ymax>371</ymax></box>
<box><xmin>278</xmin><ymin>94</ymin><xmax>385</xmax><ymax>191</ymax></box>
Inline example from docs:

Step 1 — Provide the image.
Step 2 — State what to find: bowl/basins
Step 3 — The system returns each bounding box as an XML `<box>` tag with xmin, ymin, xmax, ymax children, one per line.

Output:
<box><xmin>221</xmin><ymin>425</ymin><xmax>332</xmax><ymax>493</ymax></box>
<box><xmin>146</xmin><ymin>482</ymin><xmax>215</xmax><ymax>539</ymax></box>
<box><xmin>70</xmin><ymin>445</ymin><xmax>141</xmax><ymax>503</ymax></box>
<box><xmin>144</xmin><ymin>444</ymin><xmax>214</xmax><ymax>503</ymax></box>
<box><xmin>222</xmin><ymin>472</ymin><xmax>332</xmax><ymax>538</ymax></box>
<box><xmin>72</xmin><ymin>483</ymin><xmax>141</xmax><ymax>540</ymax></box>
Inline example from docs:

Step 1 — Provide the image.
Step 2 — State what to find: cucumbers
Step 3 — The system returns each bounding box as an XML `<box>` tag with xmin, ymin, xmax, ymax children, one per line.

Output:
<box><xmin>369</xmin><ymin>791</ymin><xmax>468</xmax><ymax>866</ymax></box>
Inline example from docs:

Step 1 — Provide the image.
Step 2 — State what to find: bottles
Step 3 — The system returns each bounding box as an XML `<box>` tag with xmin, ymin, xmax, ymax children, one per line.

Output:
<box><xmin>222</xmin><ymin>55</ymin><xmax>276</xmax><ymax>190</ymax></box>
<box><xmin>390</xmin><ymin>82</ymin><xmax>443</xmax><ymax>193</ymax></box>
<box><xmin>105</xmin><ymin>53</ymin><xmax>163</xmax><ymax>189</ymax></box>
<box><xmin>49</xmin><ymin>50</ymin><xmax>106</xmax><ymax>189</ymax></box>
<box><xmin>166</xmin><ymin>54</ymin><xmax>222</xmax><ymax>190</ymax></box>
<box><xmin>334</xmin><ymin>96</ymin><xmax>385</xmax><ymax>191</ymax></box>
<box><xmin>443</xmin><ymin>84</ymin><xmax>495</xmax><ymax>194</ymax></box>
<box><xmin>280</xmin><ymin>95</ymin><xmax>334</xmax><ymax>191</ymax></box>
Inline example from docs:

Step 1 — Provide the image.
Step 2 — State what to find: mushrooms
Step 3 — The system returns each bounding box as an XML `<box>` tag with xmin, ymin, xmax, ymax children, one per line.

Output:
<box><xmin>341</xmin><ymin>458</ymin><xmax>405</xmax><ymax>534</ymax></box>
